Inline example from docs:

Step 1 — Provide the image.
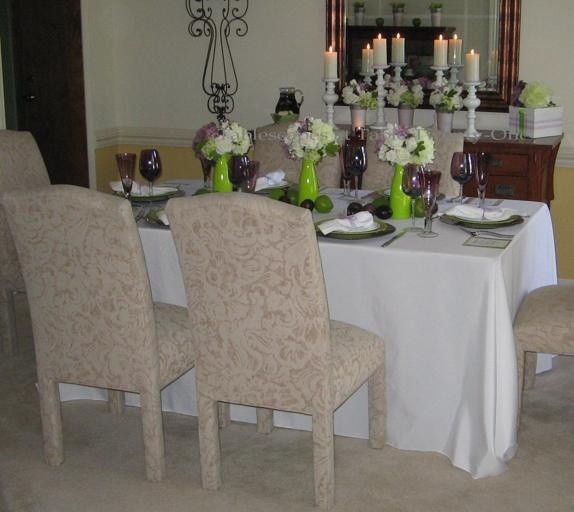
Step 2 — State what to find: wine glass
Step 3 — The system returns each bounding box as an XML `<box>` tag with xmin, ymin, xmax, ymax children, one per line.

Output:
<box><xmin>227</xmin><ymin>154</ymin><xmax>260</xmax><ymax>193</ymax></box>
<box><xmin>335</xmin><ymin>143</ymin><xmax>355</xmax><ymax>202</ymax></box>
<box><xmin>114</xmin><ymin>151</ymin><xmax>137</xmax><ymax>202</ymax></box>
<box><xmin>138</xmin><ymin>148</ymin><xmax>162</xmax><ymax>208</ymax></box>
<box><xmin>416</xmin><ymin>171</ymin><xmax>441</xmax><ymax>239</ymax></box>
<box><xmin>400</xmin><ymin>163</ymin><xmax>426</xmax><ymax>236</ymax></box>
<box><xmin>450</xmin><ymin>151</ymin><xmax>472</xmax><ymax>205</ymax></box>
<box><xmin>344</xmin><ymin>144</ymin><xmax>368</xmax><ymax>205</ymax></box>
<box><xmin>472</xmin><ymin>153</ymin><xmax>493</xmax><ymax>209</ymax></box>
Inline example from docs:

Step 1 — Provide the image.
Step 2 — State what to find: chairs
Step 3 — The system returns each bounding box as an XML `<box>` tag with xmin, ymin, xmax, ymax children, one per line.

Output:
<box><xmin>360</xmin><ymin>127</ymin><xmax>468</xmax><ymax>199</ymax></box>
<box><xmin>252</xmin><ymin>125</ymin><xmax>342</xmax><ymax>190</ymax></box>
<box><xmin>0</xmin><ymin>121</ymin><xmax>56</xmax><ymax>357</ymax></box>
<box><xmin>2</xmin><ymin>184</ymin><xmax>199</xmax><ymax>489</ymax></box>
<box><xmin>160</xmin><ymin>187</ymin><xmax>386</xmax><ymax>512</ymax></box>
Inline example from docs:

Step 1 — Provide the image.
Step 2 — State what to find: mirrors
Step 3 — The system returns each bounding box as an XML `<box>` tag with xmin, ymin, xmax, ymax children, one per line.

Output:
<box><xmin>321</xmin><ymin>0</ymin><xmax>522</xmax><ymax>114</ymax></box>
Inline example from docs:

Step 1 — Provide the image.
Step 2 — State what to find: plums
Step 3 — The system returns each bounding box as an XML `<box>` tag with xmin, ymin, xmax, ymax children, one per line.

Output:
<box><xmin>278</xmin><ymin>196</ymin><xmax>290</xmax><ymax>204</ymax></box>
<box><xmin>363</xmin><ymin>204</ymin><xmax>376</xmax><ymax>215</ymax></box>
<box><xmin>426</xmin><ymin>199</ymin><xmax>438</xmax><ymax>213</ymax></box>
<box><xmin>346</xmin><ymin>202</ymin><xmax>362</xmax><ymax>215</ymax></box>
<box><xmin>375</xmin><ymin>205</ymin><xmax>394</xmax><ymax>219</ymax></box>
<box><xmin>299</xmin><ymin>199</ymin><xmax>315</xmax><ymax>211</ymax></box>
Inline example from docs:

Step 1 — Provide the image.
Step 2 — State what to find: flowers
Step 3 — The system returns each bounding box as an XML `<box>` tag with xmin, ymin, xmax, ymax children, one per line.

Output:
<box><xmin>193</xmin><ymin>121</ymin><xmax>250</xmax><ymax>157</ymax></box>
<box><xmin>428</xmin><ymin>84</ymin><xmax>466</xmax><ymax>113</ymax></box>
<box><xmin>383</xmin><ymin>80</ymin><xmax>424</xmax><ymax>107</ymax></box>
<box><xmin>339</xmin><ymin>76</ymin><xmax>380</xmax><ymax>113</ymax></box>
<box><xmin>281</xmin><ymin>117</ymin><xmax>343</xmax><ymax>156</ymax></box>
<box><xmin>375</xmin><ymin>121</ymin><xmax>438</xmax><ymax>168</ymax></box>
<box><xmin>510</xmin><ymin>75</ymin><xmax>556</xmax><ymax>108</ymax></box>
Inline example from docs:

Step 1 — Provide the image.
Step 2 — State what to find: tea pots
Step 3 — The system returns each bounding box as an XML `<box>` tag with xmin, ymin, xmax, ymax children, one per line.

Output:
<box><xmin>275</xmin><ymin>85</ymin><xmax>304</xmax><ymax>121</ymax></box>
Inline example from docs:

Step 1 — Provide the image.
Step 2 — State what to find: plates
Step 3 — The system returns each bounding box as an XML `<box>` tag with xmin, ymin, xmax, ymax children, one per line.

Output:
<box><xmin>109</xmin><ymin>186</ymin><xmax>186</xmax><ymax>201</ymax></box>
<box><xmin>441</xmin><ymin>213</ymin><xmax>523</xmax><ymax>229</ymax></box>
<box><xmin>313</xmin><ymin>218</ymin><xmax>395</xmax><ymax>241</ymax></box>
<box><xmin>377</xmin><ymin>185</ymin><xmax>445</xmax><ymax>202</ymax></box>
<box><xmin>266</xmin><ymin>179</ymin><xmax>296</xmax><ymax>189</ymax></box>
<box><xmin>143</xmin><ymin>209</ymin><xmax>169</xmax><ymax>229</ymax></box>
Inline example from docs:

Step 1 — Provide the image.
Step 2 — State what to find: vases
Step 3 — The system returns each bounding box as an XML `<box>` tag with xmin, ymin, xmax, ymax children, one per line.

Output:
<box><xmin>387</xmin><ymin>164</ymin><xmax>415</xmax><ymax>221</ymax></box>
<box><xmin>293</xmin><ymin>158</ymin><xmax>320</xmax><ymax>212</ymax></box>
<box><xmin>436</xmin><ymin>110</ymin><xmax>452</xmax><ymax>135</ymax></box>
<box><xmin>349</xmin><ymin>103</ymin><xmax>366</xmax><ymax>132</ymax></box>
<box><xmin>209</xmin><ymin>151</ymin><xmax>235</xmax><ymax>193</ymax></box>
<box><xmin>397</xmin><ymin>104</ymin><xmax>416</xmax><ymax>128</ymax></box>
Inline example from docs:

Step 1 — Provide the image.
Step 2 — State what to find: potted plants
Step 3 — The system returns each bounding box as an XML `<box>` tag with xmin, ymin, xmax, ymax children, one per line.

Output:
<box><xmin>389</xmin><ymin>0</ymin><xmax>406</xmax><ymax>27</ymax></box>
<box><xmin>429</xmin><ymin>2</ymin><xmax>443</xmax><ymax>27</ymax></box>
<box><xmin>350</xmin><ymin>2</ymin><xmax>367</xmax><ymax>28</ymax></box>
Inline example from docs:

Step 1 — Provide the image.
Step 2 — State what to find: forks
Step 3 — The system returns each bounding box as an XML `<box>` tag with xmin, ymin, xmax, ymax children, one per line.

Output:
<box><xmin>457</xmin><ymin>226</ymin><xmax>513</xmax><ymax>240</ymax></box>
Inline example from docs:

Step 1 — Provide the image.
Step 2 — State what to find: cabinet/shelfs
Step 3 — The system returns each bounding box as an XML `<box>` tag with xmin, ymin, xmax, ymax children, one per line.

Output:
<box><xmin>463</xmin><ymin>129</ymin><xmax>562</xmax><ymax>208</ymax></box>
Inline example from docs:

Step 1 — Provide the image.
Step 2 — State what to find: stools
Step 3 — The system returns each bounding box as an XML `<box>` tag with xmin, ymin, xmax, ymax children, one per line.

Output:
<box><xmin>514</xmin><ymin>282</ymin><xmax>573</xmax><ymax>435</ymax></box>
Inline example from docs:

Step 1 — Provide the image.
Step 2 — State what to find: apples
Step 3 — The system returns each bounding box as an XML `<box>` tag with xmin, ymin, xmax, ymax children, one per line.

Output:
<box><xmin>411</xmin><ymin>198</ymin><xmax>425</xmax><ymax>217</ymax></box>
<box><xmin>287</xmin><ymin>190</ymin><xmax>299</xmax><ymax>205</ymax></box>
<box><xmin>270</xmin><ymin>189</ymin><xmax>283</xmax><ymax>202</ymax></box>
<box><xmin>315</xmin><ymin>195</ymin><xmax>333</xmax><ymax>213</ymax></box>
<box><xmin>195</xmin><ymin>189</ymin><xmax>208</xmax><ymax>196</ymax></box>
<box><xmin>413</xmin><ymin>18</ymin><xmax>421</xmax><ymax>26</ymax></box>
<box><xmin>375</xmin><ymin>18</ymin><xmax>384</xmax><ymax>26</ymax></box>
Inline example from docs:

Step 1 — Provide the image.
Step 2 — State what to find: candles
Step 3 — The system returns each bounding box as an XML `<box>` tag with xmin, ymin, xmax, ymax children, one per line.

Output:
<box><xmin>391</xmin><ymin>32</ymin><xmax>405</xmax><ymax>64</ymax></box>
<box><xmin>361</xmin><ymin>44</ymin><xmax>373</xmax><ymax>75</ymax></box>
<box><xmin>372</xmin><ymin>33</ymin><xmax>388</xmax><ymax>67</ymax></box>
<box><xmin>433</xmin><ymin>33</ymin><xmax>449</xmax><ymax>68</ymax></box>
<box><xmin>322</xmin><ymin>46</ymin><xmax>338</xmax><ymax>79</ymax></box>
<box><xmin>448</xmin><ymin>33</ymin><xmax>462</xmax><ymax>65</ymax></box>
<box><xmin>464</xmin><ymin>47</ymin><xmax>482</xmax><ymax>85</ymax></box>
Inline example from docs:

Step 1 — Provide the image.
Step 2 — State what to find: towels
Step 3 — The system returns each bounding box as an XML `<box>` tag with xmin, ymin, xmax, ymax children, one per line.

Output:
<box><xmin>110</xmin><ymin>176</ymin><xmax>181</xmax><ymax>202</ymax></box>
<box><xmin>318</xmin><ymin>209</ymin><xmax>381</xmax><ymax>236</ymax></box>
<box><xmin>444</xmin><ymin>200</ymin><xmax>529</xmax><ymax>225</ymax></box>
<box><xmin>250</xmin><ymin>170</ymin><xmax>294</xmax><ymax>195</ymax></box>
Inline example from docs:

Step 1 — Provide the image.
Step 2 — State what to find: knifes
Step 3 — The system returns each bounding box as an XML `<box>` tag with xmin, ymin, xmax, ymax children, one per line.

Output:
<box><xmin>380</xmin><ymin>229</ymin><xmax>409</xmax><ymax>248</ymax></box>
<box><xmin>360</xmin><ymin>187</ymin><xmax>389</xmax><ymax>199</ymax></box>
